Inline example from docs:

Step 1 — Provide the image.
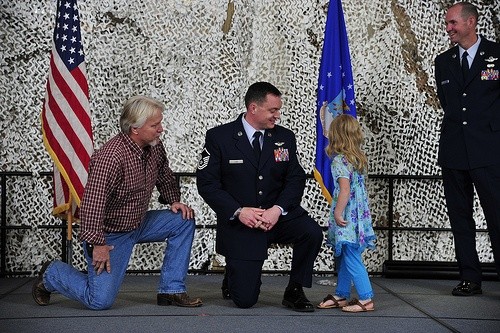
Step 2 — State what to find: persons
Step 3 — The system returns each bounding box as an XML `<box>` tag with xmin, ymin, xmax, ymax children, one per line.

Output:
<box><xmin>435</xmin><ymin>2</ymin><xmax>500</xmax><ymax>295</ymax></box>
<box><xmin>32</xmin><ymin>95</ymin><xmax>203</xmax><ymax>311</ymax></box>
<box><xmin>316</xmin><ymin>114</ymin><xmax>376</xmax><ymax>312</ymax></box>
<box><xmin>195</xmin><ymin>82</ymin><xmax>323</xmax><ymax>311</ymax></box>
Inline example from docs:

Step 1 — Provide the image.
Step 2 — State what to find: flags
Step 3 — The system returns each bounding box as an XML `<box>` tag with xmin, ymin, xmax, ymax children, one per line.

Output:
<box><xmin>38</xmin><ymin>0</ymin><xmax>94</xmax><ymax>224</ymax></box>
<box><xmin>313</xmin><ymin>0</ymin><xmax>356</xmax><ymax>206</ymax></box>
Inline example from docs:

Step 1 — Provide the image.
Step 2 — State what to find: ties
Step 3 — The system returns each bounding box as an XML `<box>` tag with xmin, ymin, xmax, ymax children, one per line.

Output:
<box><xmin>252</xmin><ymin>131</ymin><xmax>262</xmax><ymax>162</ymax></box>
<box><xmin>462</xmin><ymin>51</ymin><xmax>469</xmax><ymax>78</ymax></box>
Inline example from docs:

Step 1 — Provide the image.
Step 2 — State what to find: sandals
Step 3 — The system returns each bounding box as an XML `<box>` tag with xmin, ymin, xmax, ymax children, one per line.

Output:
<box><xmin>316</xmin><ymin>294</ymin><xmax>347</xmax><ymax>309</ymax></box>
<box><xmin>342</xmin><ymin>298</ymin><xmax>374</xmax><ymax>311</ymax></box>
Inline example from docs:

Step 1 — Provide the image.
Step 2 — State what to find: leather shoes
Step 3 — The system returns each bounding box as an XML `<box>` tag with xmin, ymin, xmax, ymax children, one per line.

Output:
<box><xmin>157</xmin><ymin>292</ymin><xmax>203</xmax><ymax>307</ymax></box>
<box><xmin>32</xmin><ymin>261</ymin><xmax>51</xmax><ymax>306</ymax></box>
<box><xmin>282</xmin><ymin>286</ymin><xmax>315</xmax><ymax>312</ymax></box>
<box><xmin>452</xmin><ymin>281</ymin><xmax>483</xmax><ymax>296</ymax></box>
<box><xmin>222</xmin><ymin>279</ymin><xmax>231</xmax><ymax>299</ymax></box>
